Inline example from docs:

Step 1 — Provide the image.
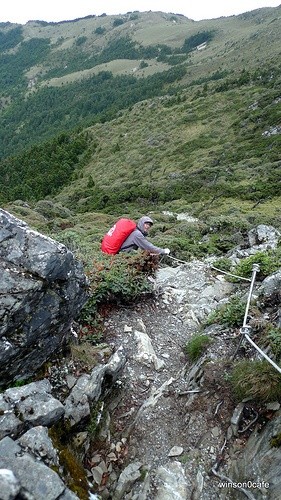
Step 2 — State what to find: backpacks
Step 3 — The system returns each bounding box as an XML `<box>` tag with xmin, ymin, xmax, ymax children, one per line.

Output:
<box><xmin>101</xmin><ymin>218</ymin><xmax>144</xmax><ymax>255</ymax></box>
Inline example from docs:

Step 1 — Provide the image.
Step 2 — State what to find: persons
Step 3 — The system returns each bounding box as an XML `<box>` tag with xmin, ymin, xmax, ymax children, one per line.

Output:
<box><xmin>115</xmin><ymin>216</ymin><xmax>170</xmax><ymax>256</ymax></box>
